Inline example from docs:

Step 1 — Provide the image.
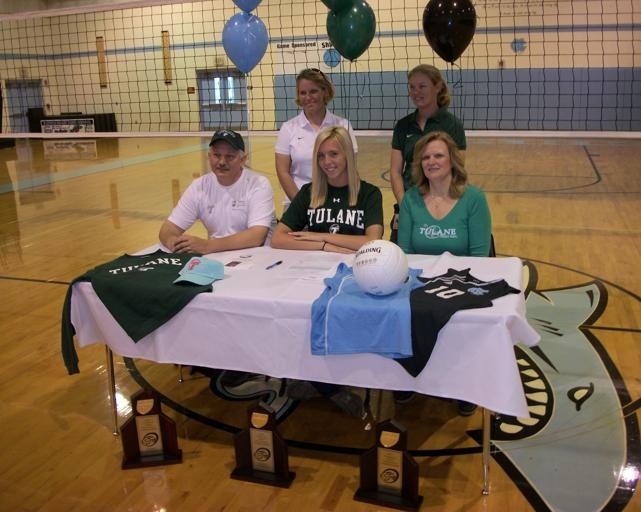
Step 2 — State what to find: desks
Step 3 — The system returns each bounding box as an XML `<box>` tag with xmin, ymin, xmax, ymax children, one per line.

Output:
<box><xmin>72</xmin><ymin>242</ymin><xmax>532</xmax><ymax>496</ymax></box>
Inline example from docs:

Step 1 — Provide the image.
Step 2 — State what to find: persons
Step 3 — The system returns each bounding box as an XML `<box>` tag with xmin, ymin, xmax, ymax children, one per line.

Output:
<box><xmin>390</xmin><ymin>65</ymin><xmax>468</xmax><ymax>249</ymax></box>
<box><xmin>158</xmin><ymin>127</ymin><xmax>276</xmax><ymax>255</ymax></box>
<box><xmin>276</xmin><ymin>69</ymin><xmax>357</xmax><ymax>211</ymax></box>
<box><xmin>270</xmin><ymin>126</ymin><xmax>385</xmax><ymax>416</ymax></box>
<box><xmin>396</xmin><ymin>132</ymin><xmax>494</xmax><ymax>416</ymax></box>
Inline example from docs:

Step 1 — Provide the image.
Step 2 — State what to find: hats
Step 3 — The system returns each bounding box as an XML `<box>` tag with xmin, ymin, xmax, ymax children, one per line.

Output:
<box><xmin>172</xmin><ymin>255</ymin><xmax>225</xmax><ymax>286</ymax></box>
<box><xmin>207</xmin><ymin>125</ymin><xmax>248</xmax><ymax>153</ymax></box>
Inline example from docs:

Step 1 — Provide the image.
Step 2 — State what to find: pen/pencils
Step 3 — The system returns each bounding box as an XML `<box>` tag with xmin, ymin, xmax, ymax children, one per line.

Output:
<box><xmin>265</xmin><ymin>261</ymin><xmax>282</xmax><ymax>270</ymax></box>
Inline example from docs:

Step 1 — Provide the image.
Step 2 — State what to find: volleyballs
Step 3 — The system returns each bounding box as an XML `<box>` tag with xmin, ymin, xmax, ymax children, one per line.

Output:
<box><xmin>353</xmin><ymin>239</ymin><xmax>409</xmax><ymax>296</ymax></box>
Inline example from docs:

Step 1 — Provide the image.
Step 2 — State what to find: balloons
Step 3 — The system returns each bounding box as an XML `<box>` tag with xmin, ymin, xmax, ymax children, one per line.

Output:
<box><xmin>323</xmin><ymin>1</ymin><xmax>354</xmax><ymax>15</ymax></box>
<box><xmin>424</xmin><ymin>1</ymin><xmax>477</xmax><ymax>65</ymax></box>
<box><xmin>223</xmin><ymin>15</ymin><xmax>269</xmax><ymax>77</ymax></box>
<box><xmin>234</xmin><ymin>0</ymin><xmax>260</xmax><ymax>16</ymax></box>
<box><xmin>327</xmin><ymin>0</ymin><xmax>376</xmax><ymax>65</ymax></box>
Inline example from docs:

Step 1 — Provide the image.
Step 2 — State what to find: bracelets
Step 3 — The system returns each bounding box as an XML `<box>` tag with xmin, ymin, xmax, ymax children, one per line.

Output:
<box><xmin>320</xmin><ymin>243</ymin><xmax>329</xmax><ymax>252</ymax></box>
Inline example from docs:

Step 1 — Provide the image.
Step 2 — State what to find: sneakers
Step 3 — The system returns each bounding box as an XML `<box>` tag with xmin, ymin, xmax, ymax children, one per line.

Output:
<box><xmin>457</xmin><ymin>400</ymin><xmax>479</xmax><ymax>418</ymax></box>
<box><xmin>391</xmin><ymin>389</ymin><xmax>417</xmax><ymax>405</ymax></box>
